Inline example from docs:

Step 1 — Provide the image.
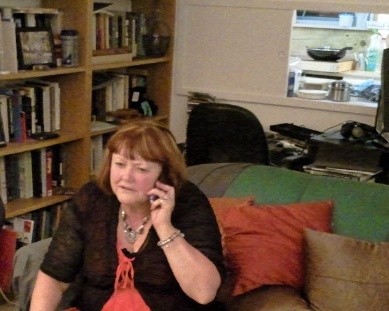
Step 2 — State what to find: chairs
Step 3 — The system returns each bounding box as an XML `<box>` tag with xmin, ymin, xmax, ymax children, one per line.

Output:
<box><xmin>186</xmin><ymin>103</ymin><xmax>268</xmax><ymax>165</ymax></box>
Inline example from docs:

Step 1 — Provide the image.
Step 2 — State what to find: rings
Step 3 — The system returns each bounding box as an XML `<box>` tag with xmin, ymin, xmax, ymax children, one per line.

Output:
<box><xmin>163</xmin><ymin>194</ymin><xmax>170</xmax><ymax>199</ymax></box>
<box><xmin>160</xmin><ymin>200</ymin><xmax>167</xmax><ymax>204</ymax></box>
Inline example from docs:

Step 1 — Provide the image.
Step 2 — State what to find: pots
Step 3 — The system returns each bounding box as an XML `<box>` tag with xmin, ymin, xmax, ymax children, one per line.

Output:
<box><xmin>306</xmin><ymin>46</ymin><xmax>353</xmax><ymax>61</ymax></box>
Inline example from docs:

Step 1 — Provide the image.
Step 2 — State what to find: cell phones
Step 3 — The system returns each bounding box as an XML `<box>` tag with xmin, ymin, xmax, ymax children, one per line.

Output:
<box><xmin>149</xmin><ymin>171</ymin><xmax>170</xmax><ymax>204</ymax></box>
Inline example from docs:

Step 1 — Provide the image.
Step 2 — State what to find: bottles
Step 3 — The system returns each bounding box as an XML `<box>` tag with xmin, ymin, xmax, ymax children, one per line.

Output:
<box><xmin>60</xmin><ymin>30</ymin><xmax>80</xmax><ymax>68</ymax></box>
<box><xmin>330</xmin><ymin>81</ymin><xmax>350</xmax><ymax>102</ymax></box>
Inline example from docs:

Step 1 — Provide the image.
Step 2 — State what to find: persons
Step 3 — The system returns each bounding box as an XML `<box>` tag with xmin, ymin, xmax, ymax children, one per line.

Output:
<box><xmin>30</xmin><ymin>121</ymin><xmax>224</xmax><ymax>311</ymax></box>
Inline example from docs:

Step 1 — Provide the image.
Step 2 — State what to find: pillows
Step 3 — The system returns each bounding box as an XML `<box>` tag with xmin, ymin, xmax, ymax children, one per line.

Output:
<box><xmin>208</xmin><ymin>197</ymin><xmax>389</xmax><ymax>311</ymax></box>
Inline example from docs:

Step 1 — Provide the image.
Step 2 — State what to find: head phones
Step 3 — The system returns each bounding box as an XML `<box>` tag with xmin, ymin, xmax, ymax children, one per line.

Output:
<box><xmin>341</xmin><ymin>121</ymin><xmax>382</xmax><ymax>139</ymax></box>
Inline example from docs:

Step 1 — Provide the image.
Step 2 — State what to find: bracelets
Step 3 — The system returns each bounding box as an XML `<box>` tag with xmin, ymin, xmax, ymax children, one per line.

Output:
<box><xmin>157</xmin><ymin>229</ymin><xmax>181</xmax><ymax>246</ymax></box>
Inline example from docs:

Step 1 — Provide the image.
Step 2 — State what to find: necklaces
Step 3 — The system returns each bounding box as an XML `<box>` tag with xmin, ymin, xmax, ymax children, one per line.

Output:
<box><xmin>121</xmin><ymin>206</ymin><xmax>150</xmax><ymax>243</ymax></box>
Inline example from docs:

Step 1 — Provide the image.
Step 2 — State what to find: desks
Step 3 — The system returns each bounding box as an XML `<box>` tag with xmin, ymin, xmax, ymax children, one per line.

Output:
<box><xmin>265</xmin><ymin>131</ymin><xmax>389</xmax><ymax>186</ymax></box>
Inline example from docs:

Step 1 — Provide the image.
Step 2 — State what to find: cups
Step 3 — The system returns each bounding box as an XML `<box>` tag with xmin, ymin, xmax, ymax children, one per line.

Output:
<box><xmin>339</xmin><ymin>14</ymin><xmax>353</xmax><ymax>26</ymax></box>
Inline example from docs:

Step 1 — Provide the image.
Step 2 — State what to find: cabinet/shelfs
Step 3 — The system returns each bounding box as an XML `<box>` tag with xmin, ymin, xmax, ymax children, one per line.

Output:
<box><xmin>0</xmin><ymin>0</ymin><xmax>176</xmax><ymax>218</ymax></box>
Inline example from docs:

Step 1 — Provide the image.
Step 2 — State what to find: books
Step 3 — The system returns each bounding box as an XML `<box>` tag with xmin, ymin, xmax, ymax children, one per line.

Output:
<box><xmin>2</xmin><ymin>201</ymin><xmax>67</xmax><ymax>251</ymax></box>
<box><xmin>0</xmin><ymin>145</ymin><xmax>64</xmax><ymax>204</ymax></box>
<box><xmin>91</xmin><ymin>67</ymin><xmax>148</xmax><ymax>122</ymax></box>
<box><xmin>0</xmin><ymin>79</ymin><xmax>61</xmax><ymax>143</ymax></box>
<box><xmin>0</xmin><ymin>5</ymin><xmax>66</xmax><ymax>73</ymax></box>
<box><xmin>91</xmin><ymin>133</ymin><xmax>116</xmax><ymax>171</ymax></box>
<box><xmin>91</xmin><ymin>2</ymin><xmax>149</xmax><ymax>64</ymax></box>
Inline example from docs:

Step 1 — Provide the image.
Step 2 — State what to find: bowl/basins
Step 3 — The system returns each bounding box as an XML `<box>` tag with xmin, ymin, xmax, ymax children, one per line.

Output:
<box><xmin>141</xmin><ymin>34</ymin><xmax>170</xmax><ymax>58</ymax></box>
<box><xmin>296</xmin><ymin>90</ymin><xmax>329</xmax><ymax>100</ymax></box>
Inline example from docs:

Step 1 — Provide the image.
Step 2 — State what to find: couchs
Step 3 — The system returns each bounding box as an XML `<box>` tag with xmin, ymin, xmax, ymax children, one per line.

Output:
<box><xmin>14</xmin><ymin>163</ymin><xmax>389</xmax><ymax>311</ymax></box>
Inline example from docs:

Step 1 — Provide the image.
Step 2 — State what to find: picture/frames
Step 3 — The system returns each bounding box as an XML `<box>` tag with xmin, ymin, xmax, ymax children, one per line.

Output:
<box><xmin>15</xmin><ymin>27</ymin><xmax>56</xmax><ymax>70</ymax></box>
